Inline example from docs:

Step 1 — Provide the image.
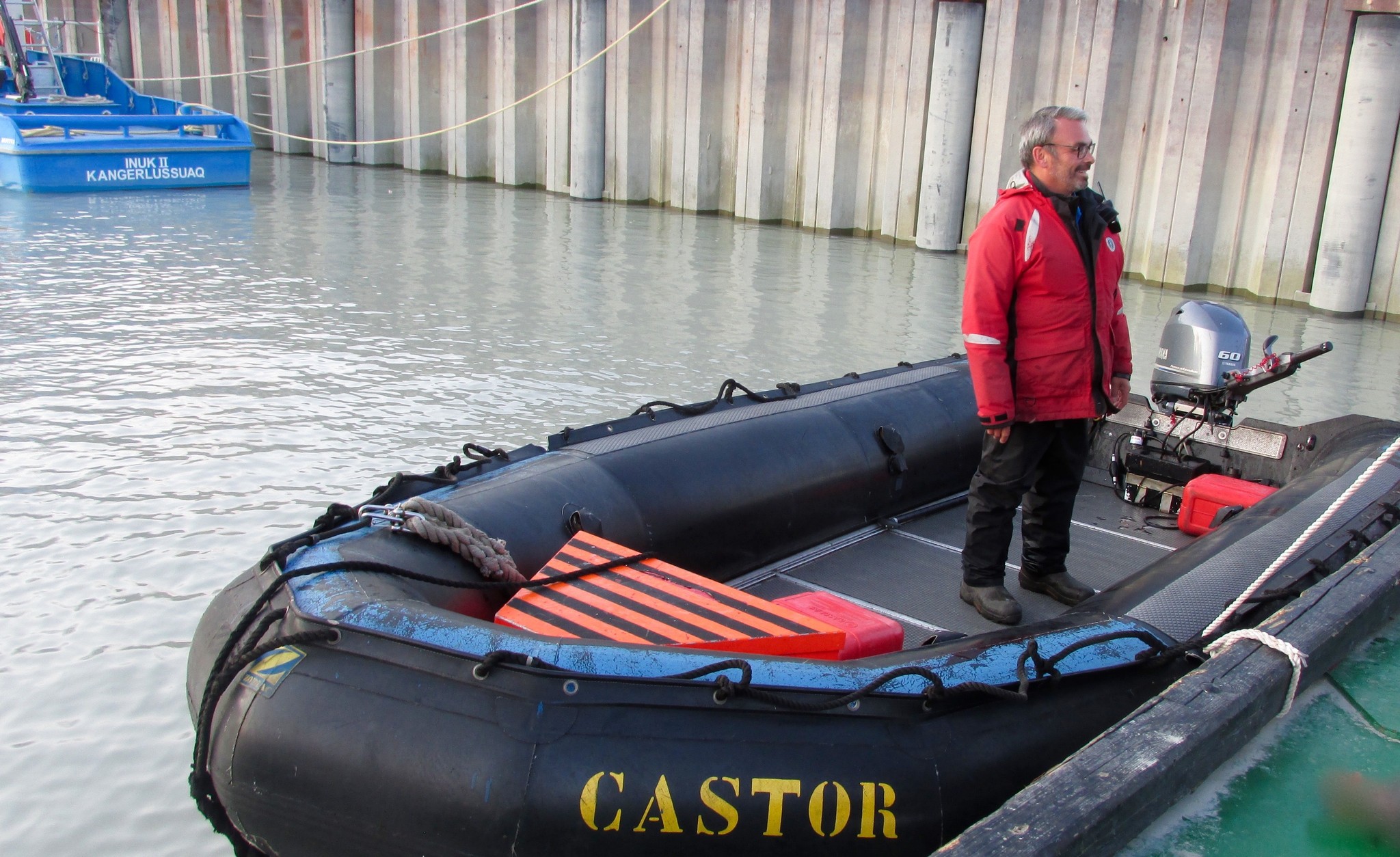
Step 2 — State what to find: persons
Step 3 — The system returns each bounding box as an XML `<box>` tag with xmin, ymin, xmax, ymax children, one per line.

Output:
<box><xmin>959</xmin><ymin>106</ymin><xmax>1134</xmax><ymax>625</ymax></box>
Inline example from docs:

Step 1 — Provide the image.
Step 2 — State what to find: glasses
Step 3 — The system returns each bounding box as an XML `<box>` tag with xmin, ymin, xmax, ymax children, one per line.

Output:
<box><xmin>1038</xmin><ymin>142</ymin><xmax>1096</xmax><ymax>159</ymax></box>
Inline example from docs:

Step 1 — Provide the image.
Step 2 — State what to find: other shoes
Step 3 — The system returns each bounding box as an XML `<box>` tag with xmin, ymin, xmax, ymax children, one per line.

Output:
<box><xmin>959</xmin><ymin>579</ymin><xmax>1023</xmax><ymax>624</ymax></box>
<box><xmin>1018</xmin><ymin>566</ymin><xmax>1095</xmax><ymax>607</ymax></box>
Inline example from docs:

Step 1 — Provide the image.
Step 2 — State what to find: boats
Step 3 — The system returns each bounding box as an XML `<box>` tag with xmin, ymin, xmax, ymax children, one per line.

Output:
<box><xmin>186</xmin><ymin>297</ymin><xmax>1400</xmax><ymax>856</ymax></box>
<box><xmin>0</xmin><ymin>1</ymin><xmax>256</xmax><ymax>192</ymax></box>
